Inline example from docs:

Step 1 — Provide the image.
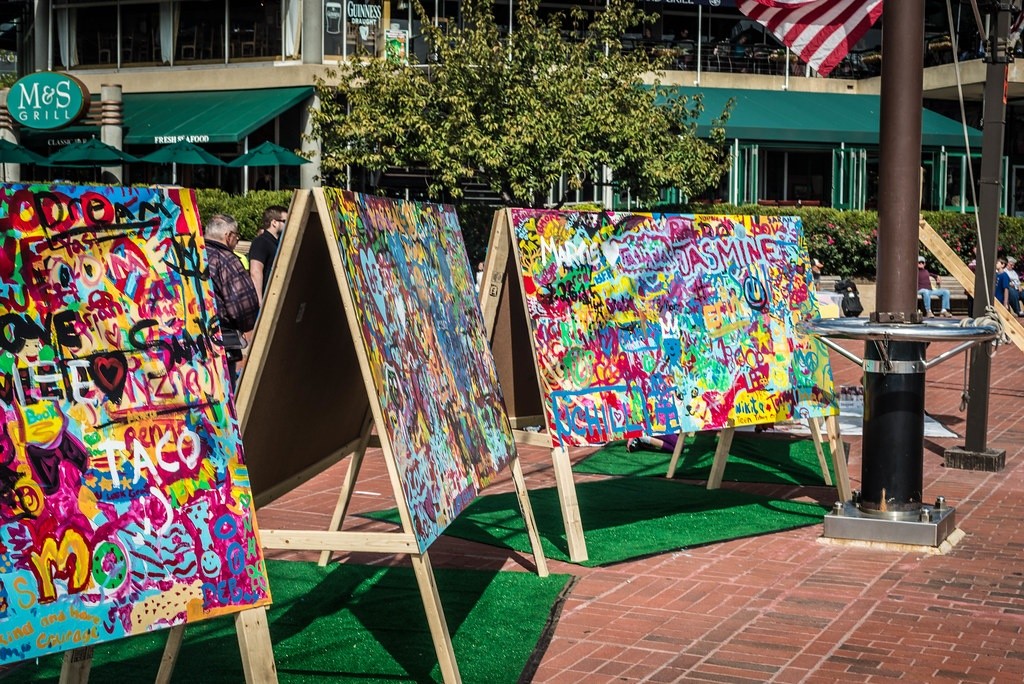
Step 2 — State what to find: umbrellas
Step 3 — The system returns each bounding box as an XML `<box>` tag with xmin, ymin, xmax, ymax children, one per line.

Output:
<box><xmin>139</xmin><ymin>140</ymin><xmax>228</xmax><ymax>167</ymax></box>
<box><xmin>228</xmin><ymin>140</ymin><xmax>313</xmax><ymax>168</ymax></box>
<box><xmin>0</xmin><ymin>138</ymin><xmax>139</xmax><ymax>168</ymax></box>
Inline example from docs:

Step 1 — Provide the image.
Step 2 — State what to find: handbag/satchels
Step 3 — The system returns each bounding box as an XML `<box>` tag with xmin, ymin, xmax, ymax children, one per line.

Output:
<box><xmin>221</xmin><ymin>323</ymin><xmax>248</xmax><ymax>362</ymax></box>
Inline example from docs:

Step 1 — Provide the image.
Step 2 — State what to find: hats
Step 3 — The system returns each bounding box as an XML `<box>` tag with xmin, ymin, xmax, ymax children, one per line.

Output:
<box><xmin>810</xmin><ymin>258</ymin><xmax>824</xmax><ymax>268</ymax></box>
<box><xmin>917</xmin><ymin>255</ymin><xmax>926</xmax><ymax>261</ymax></box>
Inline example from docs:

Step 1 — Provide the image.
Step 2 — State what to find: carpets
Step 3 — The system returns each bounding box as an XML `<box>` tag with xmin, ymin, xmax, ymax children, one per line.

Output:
<box><xmin>0</xmin><ymin>559</ymin><xmax>577</xmax><ymax>684</ymax></box>
<box><xmin>733</xmin><ymin>394</ymin><xmax>964</xmax><ymax>438</ymax></box>
<box><xmin>347</xmin><ymin>476</ymin><xmax>833</xmax><ymax>568</ymax></box>
<box><xmin>571</xmin><ymin>430</ymin><xmax>853</xmax><ymax>488</ymax></box>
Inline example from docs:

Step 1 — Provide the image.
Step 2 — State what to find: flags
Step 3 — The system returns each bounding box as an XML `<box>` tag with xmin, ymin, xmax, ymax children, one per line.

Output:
<box><xmin>735</xmin><ymin>0</ymin><xmax>884</xmax><ymax>78</ymax></box>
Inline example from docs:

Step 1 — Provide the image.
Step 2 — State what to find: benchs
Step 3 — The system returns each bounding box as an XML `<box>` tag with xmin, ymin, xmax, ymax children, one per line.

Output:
<box><xmin>820</xmin><ymin>275</ymin><xmax>855</xmax><ymax>318</ymax></box>
<box><xmin>916</xmin><ymin>276</ymin><xmax>968</xmax><ymax>299</ymax></box>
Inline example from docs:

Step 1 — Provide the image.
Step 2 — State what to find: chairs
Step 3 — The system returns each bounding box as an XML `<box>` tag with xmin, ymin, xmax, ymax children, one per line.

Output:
<box><xmin>122</xmin><ymin>28</ymin><xmax>138</xmax><ymax>62</ymax></box>
<box><xmin>151</xmin><ymin>28</ymin><xmax>161</xmax><ymax>61</ymax></box>
<box><xmin>97</xmin><ymin>32</ymin><xmax>110</xmax><ymax>64</ymax></box>
<box><xmin>200</xmin><ymin>27</ymin><xmax>218</xmax><ymax>59</ymax></box>
<box><xmin>621</xmin><ymin>31</ymin><xmax>786</xmax><ymax>74</ymax></box>
<box><xmin>220</xmin><ymin>25</ymin><xmax>238</xmax><ymax>57</ymax></box>
<box><xmin>242</xmin><ymin>22</ymin><xmax>265</xmax><ymax>57</ymax></box>
<box><xmin>182</xmin><ymin>26</ymin><xmax>199</xmax><ymax>59</ymax></box>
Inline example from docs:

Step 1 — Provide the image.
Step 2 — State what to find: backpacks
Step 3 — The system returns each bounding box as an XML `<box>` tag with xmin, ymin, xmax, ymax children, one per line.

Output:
<box><xmin>840</xmin><ymin>291</ymin><xmax>865</xmax><ymax>319</ymax></box>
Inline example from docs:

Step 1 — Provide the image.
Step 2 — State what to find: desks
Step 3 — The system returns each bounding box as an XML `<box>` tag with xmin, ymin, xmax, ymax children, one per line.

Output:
<box><xmin>105</xmin><ymin>35</ymin><xmax>132</xmax><ymax>63</ymax></box>
<box><xmin>231</xmin><ymin>29</ymin><xmax>255</xmax><ymax>57</ymax></box>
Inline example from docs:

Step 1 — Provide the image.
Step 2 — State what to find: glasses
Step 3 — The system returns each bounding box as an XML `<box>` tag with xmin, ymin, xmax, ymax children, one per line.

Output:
<box><xmin>229</xmin><ymin>231</ymin><xmax>243</xmax><ymax>241</ymax></box>
<box><xmin>275</xmin><ymin>218</ymin><xmax>286</xmax><ymax>224</ymax></box>
<box><xmin>919</xmin><ymin>261</ymin><xmax>924</xmax><ymax>264</ymax></box>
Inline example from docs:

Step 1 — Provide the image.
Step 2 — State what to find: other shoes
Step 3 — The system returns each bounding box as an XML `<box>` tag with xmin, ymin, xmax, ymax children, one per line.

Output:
<box><xmin>926</xmin><ymin>310</ymin><xmax>935</xmax><ymax>318</ymax></box>
<box><xmin>940</xmin><ymin>310</ymin><xmax>952</xmax><ymax>318</ymax></box>
<box><xmin>1017</xmin><ymin>310</ymin><xmax>1024</xmax><ymax>317</ymax></box>
<box><xmin>626</xmin><ymin>437</ymin><xmax>640</xmax><ymax>454</ymax></box>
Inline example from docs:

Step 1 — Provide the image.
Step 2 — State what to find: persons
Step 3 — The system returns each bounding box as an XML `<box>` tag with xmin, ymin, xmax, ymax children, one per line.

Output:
<box><xmin>255</xmin><ymin>174</ymin><xmax>272</xmax><ymax>191</ymax></box>
<box><xmin>669</xmin><ymin>28</ymin><xmax>690</xmax><ymax>71</ymax></box>
<box><xmin>964</xmin><ymin>245</ymin><xmax>1024</xmax><ymax>319</ymax></box>
<box><xmin>641</xmin><ymin>27</ymin><xmax>655</xmax><ymax>54</ymax></box>
<box><xmin>729</xmin><ymin>33</ymin><xmax>749</xmax><ymax>72</ymax></box>
<box><xmin>194</xmin><ymin>167</ymin><xmax>210</xmax><ymax>188</ymax></box>
<box><xmin>916</xmin><ymin>256</ymin><xmax>953</xmax><ymax>318</ymax></box>
<box><xmin>810</xmin><ymin>258</ymin><xmax>824</xmax><ymax>290</ymax></box>
<box><xmin>203</xmin><ymin>205</ymin><xmax>290</xmax><ymax>395</ymax></box>
<box><xmin>834</xmin><ymin>272</ymin><xmax>864</xmax><ymax>317</ymax></box>
